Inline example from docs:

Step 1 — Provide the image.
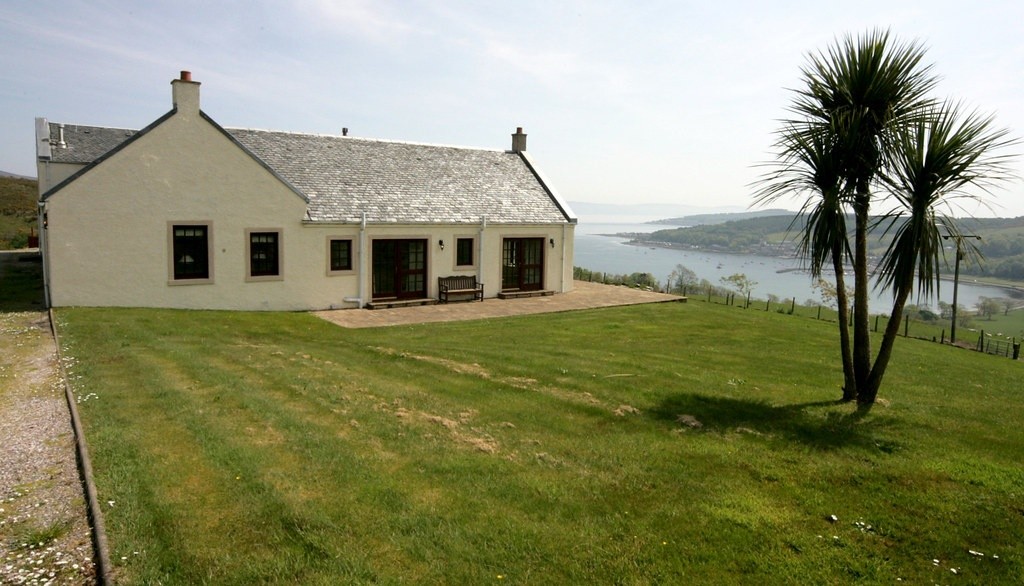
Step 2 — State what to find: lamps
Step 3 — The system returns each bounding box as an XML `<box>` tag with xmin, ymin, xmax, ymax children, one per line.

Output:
<box><xmin>43</xmin><ymin>213</ymin><xmax>47</xmax><ymax>229</ymax></box>
<box><xmin>439</xmin><ymin>240</ymin><xmax>443</xmax><ymax>250</ymax></box>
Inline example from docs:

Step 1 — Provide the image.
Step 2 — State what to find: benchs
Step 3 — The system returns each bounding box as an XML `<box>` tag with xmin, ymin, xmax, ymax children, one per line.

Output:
<box><xmin>438</xmin><ymin>275</ymin><xmax>484</xmax><ymax>303</ymax></box>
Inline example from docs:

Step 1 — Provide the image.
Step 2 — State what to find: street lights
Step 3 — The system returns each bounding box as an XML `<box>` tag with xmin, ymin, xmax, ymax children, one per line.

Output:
<box><xmin>942</xmin><ymin>235</ymin><xmax>981</xmax><ymax>343</ymax></box>
